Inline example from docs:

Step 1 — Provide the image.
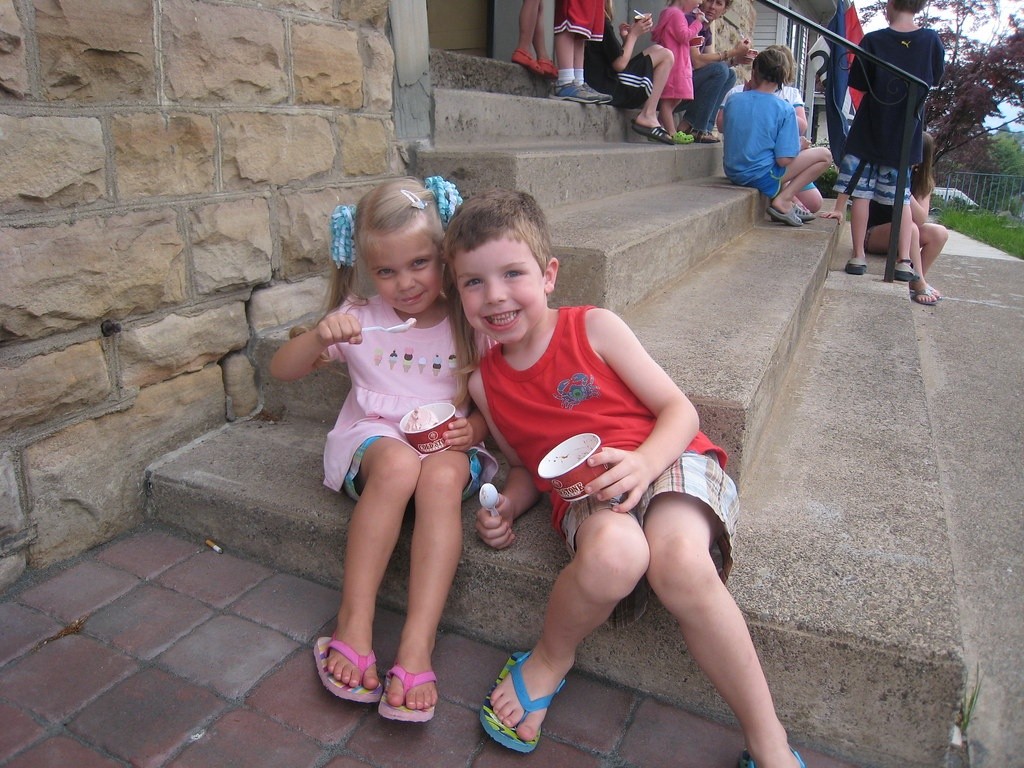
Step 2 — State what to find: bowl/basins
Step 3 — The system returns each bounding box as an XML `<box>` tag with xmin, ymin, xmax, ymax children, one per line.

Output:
<box><xmin>634</xmin><ymin>12</ymin><xmax>652</xmax><ymax>24</ymax></box>
<box><xmin>745</xmin><ymin>49</ymin><xmax>758</xmax><ymax>58</ymax></box>
<box><xmin>399</xmin><ymin>403</ymin><xmax>457</xmax><ymax>455</ymax></box>
<box><xmin>538</xmin><ymin>432</ymin><xmax>610</xmax><ymax>502</ymax></box>
<box><xmin>690</xmin><ymin>36</ymin><xmax>703</xmax><ymax>47</ymax></box>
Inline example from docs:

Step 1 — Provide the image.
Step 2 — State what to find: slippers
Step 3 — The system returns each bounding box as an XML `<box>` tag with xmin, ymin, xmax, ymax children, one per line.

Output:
<box><xmin>910</xmin><ymin>289</ymin><xmax>938</xmax><ymax>305</ymax></box>
<box><xmin>929</xmin><ymin>286</ymin><xmax>942</xmax><ymax>299</ymax></box>
<box><xmin>633</xmin><ymin>123</ymin><xmax>675</xmax><ymax>145</ymax></box>
<box><xmin>793</xmin><ymin>204</ymin><xmax>816</xmax><ymax>221</ymax></box>
<box><xmin>766</xmin><ymin>206</ymin><xmax>803</xmax><ymax>226</ymax></box>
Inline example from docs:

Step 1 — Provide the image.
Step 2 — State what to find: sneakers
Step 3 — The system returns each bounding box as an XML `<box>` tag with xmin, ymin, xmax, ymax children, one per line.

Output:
<box><xmin>548</xmin><ymin>80</ymin><xmax>602</xmax><ymax>104</ymax></box>
<box><xmin>582</xmin><ymin>82</ymin><xmax>613</xmax><ymax>104</ymax></box>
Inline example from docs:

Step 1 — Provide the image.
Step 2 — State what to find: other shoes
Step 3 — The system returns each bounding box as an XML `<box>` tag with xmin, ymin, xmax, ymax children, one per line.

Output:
<box><xmin>686</xmin><ymin>126</ymin><xmax>721</xmax><ymax>143</ymax></box>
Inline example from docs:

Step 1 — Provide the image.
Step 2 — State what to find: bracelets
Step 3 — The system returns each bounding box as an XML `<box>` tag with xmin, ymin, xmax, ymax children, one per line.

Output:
<box><xmin>724</xmin><ymin>50</ymin><xmax>728</xmax><ymax>61</ymax></box>
<box><xmin>717</xmin><ymin>51</ymin><xmax>723</xmax><ymax>61</ymax></box>
<box><xmin>730</xmin><ymin>57</ymin><xmax>737</xmax><ymax>67</ymax></box>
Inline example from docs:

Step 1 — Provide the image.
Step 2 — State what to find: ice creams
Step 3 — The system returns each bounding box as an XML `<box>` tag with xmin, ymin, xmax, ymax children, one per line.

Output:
<box><xmin>405</xmin><ymin>407</ymin><xmax>442</xmax><ymax>432</ymax></box>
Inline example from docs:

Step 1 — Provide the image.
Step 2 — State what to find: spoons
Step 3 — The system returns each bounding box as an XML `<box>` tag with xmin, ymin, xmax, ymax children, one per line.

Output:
<box><xmin>693</xmin><ymin>8</ymin><xmax>709</xmax><ymax>23</ymax></box>
<box><xmin>479</xmin><ymin>483</ymin><xmax>500</xmax><ymax>518</ymax></box>
<box><xmin>362</xmin><ymin>322</ymin><xmax>411</xmax><ymax>332</ymax></box>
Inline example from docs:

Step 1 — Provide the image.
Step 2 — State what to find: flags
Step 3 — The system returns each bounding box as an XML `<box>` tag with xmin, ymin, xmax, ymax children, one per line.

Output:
<box><xmin>806</xmin><ymin>0</ymin><xmax>865</xmax><ymax>167</ymax></box>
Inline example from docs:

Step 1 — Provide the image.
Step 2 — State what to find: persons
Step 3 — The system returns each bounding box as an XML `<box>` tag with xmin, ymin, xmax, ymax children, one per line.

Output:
<box><xmin>650</xmin><ymin>0</ymin><xmax>759</xmax><ymax>143</ymax></box>
<box><xmin>716</xmin><ymin>44</ymin><xmax>833</xmax><ymax>227</ymax></box>
<box><xmin>819</xmin><ymin>131</ymin><xmax>948</xmax><ymax>303</ymax></box>
<box><xmin>268</xmin><ymin>171</ymin><xmax>489</xmax><ymax>724</ymax></box>
<box><xmin>441</xmin><ymin>190</ymin><xmax>809</xmax><ymax>768</ymax></box>
<box><xmin>511</xmin><ymin>0</ymin><xmax>558</xmax><ymax>79</ymax></box>
<box><xmin>843</xmin><ymin>0</ymin><xmax>946</xmax><ymax>283</ymax></box>
<box><xmin>552</xmin><ymin>0</ymin><xmax>613</xmax><ymax>105</ymax></box>
<box><xmin>583</xmin><ymin>0</ymin><xmax>675</xmax><ymax>147</ymax></box>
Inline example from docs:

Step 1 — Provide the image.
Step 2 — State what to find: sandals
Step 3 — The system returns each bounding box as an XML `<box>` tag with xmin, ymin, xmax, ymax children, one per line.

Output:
<box><xmin>512</xmin><ymin>49</ymin><xmax>545</xmax><ymax>75</ymax></box>
<box><xmin>672</xmin><ymin>130</ymin><xmax>694</xmax><ymax>143</ymax></box>
<box><xmin>479</xmin><ymin>649</ymin><xmax>566</xmax><ymax>753</ymax></box>
<box><xmin>893</xmin><ymin>263</ymin><xmax>919</xmax><ymax>282</ymax></box>
<box><xmin>538</xmin><ymin>59</ymin><xmax>559</xmax><ymax>78</ymax></box>
<box><xmin>845</xmin><ymin>257</ymin><xmax>867</xmax><ymax>274</ymax></box>
<box><xmin>378</xmin><ymin>664</ymin><xmax>437</xmax><ymax>722</ymax></box>
<box><xmin>314</xmin><ymin>630</ymin><xmax>382</xmax><ymax>704</ymax></box>
<box><xmin>738</xmin><ymin>743</ymin><xmax>806</xmax><ymax>768</ymax></box>
